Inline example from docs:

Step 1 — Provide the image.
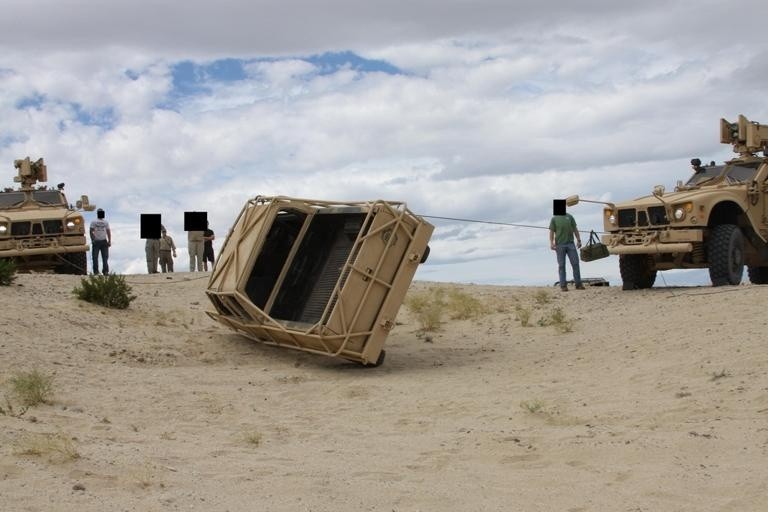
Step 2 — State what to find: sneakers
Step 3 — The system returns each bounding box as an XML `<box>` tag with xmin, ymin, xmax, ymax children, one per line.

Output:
<box><xmin>560</xmin><ymin>284</ymin><xmax>569</xmax><ymax>292</ymax></box>
<box><xmin>575</xmin><ymin>282</ymin><xmax>585</xmax><ymax>290</ymax></box>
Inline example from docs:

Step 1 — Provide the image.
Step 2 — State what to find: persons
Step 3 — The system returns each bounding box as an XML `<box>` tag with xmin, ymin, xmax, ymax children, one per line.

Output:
<box><xmin>203</xmin><ymin>224</ymin><xmax>215</xmax><ymax>270</ymax></box>
<box><xmin>550</xmin><ymin>212</ymin><xmax>585</xmax><ymax>290</ymax></box>
<box><xmin>160</xmin><ymin>228</ymin><xmax>176</xmax><ymax>273</ymax></box>
<box><xmin>145</xmin><ymin>239</ymin><xmax>160</xmax><ymax>273</ymax></box>
<box><xmin>89</xmin><ymin>209</ymin><xmax>112</xmax><ymax>275</ymax></box>
<box><xmin>188</xmin><ymin>231</ymin><xmax>204</xmax><ymax>271</ymax></box>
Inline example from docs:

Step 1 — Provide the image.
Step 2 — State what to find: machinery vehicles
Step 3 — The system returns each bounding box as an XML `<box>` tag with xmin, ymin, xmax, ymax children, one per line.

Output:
<box><xmin>563</xmin><ymin>114</ymin><xmax>766</xmax><ymax>290</ymax></box>
<box><xmin>1</xmin><ymin>156</ymin><xmax>95</xmax><ymax>279</ymax></box>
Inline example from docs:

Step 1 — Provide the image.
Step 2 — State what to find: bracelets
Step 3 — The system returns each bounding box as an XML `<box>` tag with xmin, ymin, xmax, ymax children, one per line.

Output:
<box><xmin>578</xmin><ymin>239</ymin><xmax>581</xmax><ymax>243</ymax></box>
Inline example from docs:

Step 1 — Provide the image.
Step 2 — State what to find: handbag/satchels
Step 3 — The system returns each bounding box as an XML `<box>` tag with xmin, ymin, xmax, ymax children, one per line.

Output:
<box><xmin>580</xmin><ymin>229</ymin><xmax>610</xmax><ymax>262</ymax></box>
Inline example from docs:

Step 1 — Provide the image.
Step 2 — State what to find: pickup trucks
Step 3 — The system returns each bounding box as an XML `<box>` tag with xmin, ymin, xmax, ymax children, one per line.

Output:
<box><xmin>201</xmin><ymin>194</ymin><xmax>437</xmax><ymax>367</ymax></box>
